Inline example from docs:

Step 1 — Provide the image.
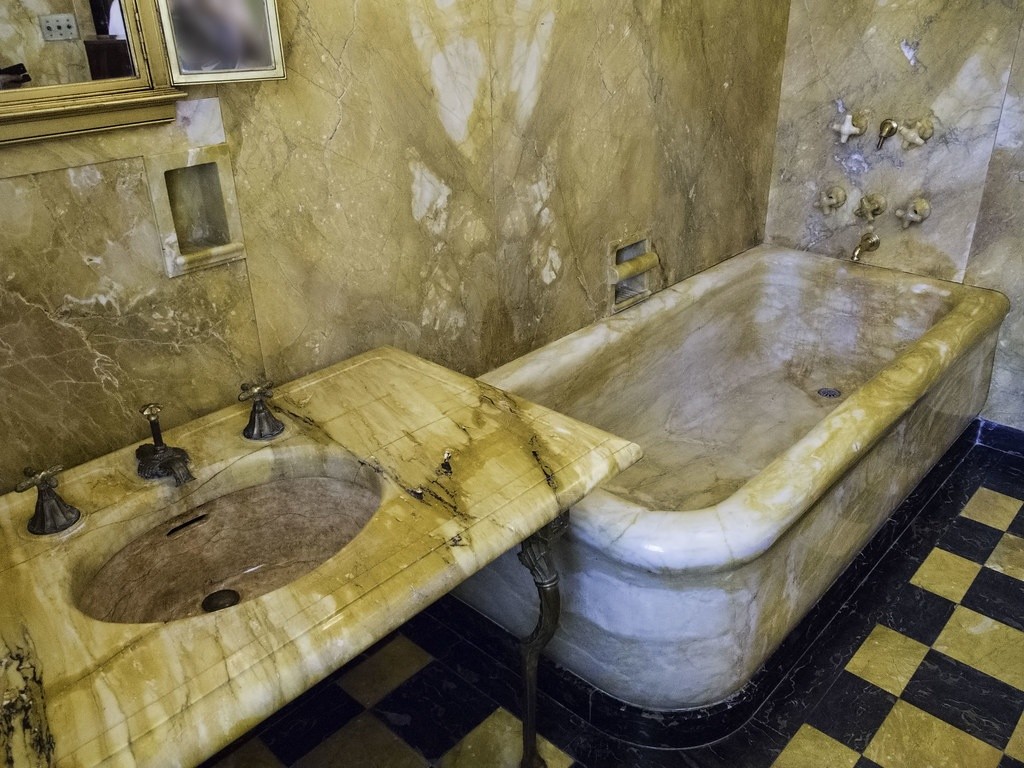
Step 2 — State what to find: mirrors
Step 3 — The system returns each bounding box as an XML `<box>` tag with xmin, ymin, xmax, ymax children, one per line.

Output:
<box><xmin>0</xmin><ymin>1</ymin><xmax>188</xmax><ymax>156</ymax></box>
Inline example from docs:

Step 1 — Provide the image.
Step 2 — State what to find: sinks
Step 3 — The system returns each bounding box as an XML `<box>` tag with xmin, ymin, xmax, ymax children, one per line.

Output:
<box><xmin>60</xmin><ymin>444</ymin><xmax>384</xmax><ymax>622</ymax></box>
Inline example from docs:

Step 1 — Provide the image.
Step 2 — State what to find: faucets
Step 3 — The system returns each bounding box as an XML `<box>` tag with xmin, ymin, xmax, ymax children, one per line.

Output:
<box><xmin>849</xmin><ymin>232</ymin><xmax>881</xmax><ymax>262</ymax></box>
<box><xmin>134</xmin><ymin>401</ymin><xmax>198</xmax><ymax>490</ymax></box>
<box><xmin>875</xmin><ymin>118</ymin><xmax>898</xmax><ymax>151</ymax></box>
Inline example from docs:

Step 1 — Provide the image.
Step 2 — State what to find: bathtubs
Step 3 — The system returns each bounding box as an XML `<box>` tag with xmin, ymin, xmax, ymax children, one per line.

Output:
<box><xmin>472</xmin><ymin>240</ymin><xmax>1011</xmax><ymax>703</ymax></box>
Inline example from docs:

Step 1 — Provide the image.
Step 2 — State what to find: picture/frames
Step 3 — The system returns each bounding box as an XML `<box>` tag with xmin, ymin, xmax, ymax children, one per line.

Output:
<box><xmin>155</xmin><ymin>2</ymin><xmax>289</xmax><ymax>92</ymax></box>
